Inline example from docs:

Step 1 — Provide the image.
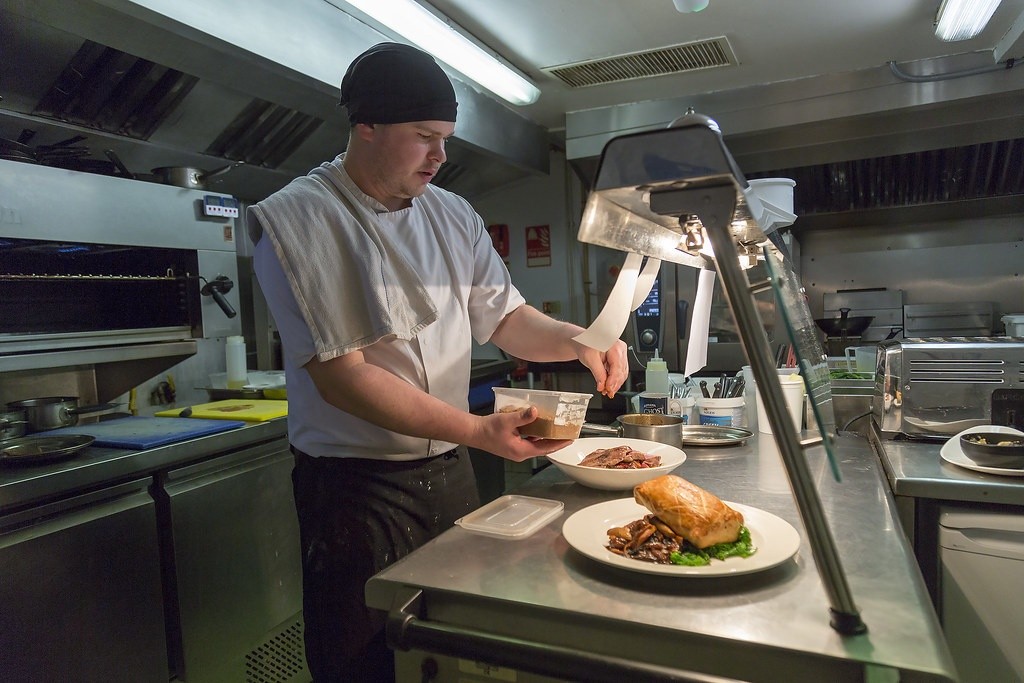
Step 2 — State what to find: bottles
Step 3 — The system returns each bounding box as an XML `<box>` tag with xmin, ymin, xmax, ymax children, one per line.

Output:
<box><xmin>646</xmin><ymin>348</ymin><xmax>668</xmax><ymax>392</ymax></box>
<box><xmin>225</xmin><ymin>336</ymin><xmax>247</xmax><ymax>390</ymax></box>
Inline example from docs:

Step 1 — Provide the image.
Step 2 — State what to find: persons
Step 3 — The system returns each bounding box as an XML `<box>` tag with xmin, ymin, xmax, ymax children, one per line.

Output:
<box><xmin>246</xmin><ymin>43</ymin><xmax>629</xmax><ymax>683</ymax></box>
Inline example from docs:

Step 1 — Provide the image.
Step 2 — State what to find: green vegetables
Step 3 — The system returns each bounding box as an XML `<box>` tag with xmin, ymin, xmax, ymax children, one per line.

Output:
<box><xmin>830</xmin><ymin>369</ymin><xmax>868</xmax><ymax>379</ymax></box>
<box><xmin>670</xmin><ymin>528</ymin><xmax>758</xmax><ymax>566</ymax></box>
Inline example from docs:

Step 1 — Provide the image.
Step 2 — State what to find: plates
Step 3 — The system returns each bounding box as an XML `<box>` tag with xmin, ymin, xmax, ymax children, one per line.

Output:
<box><xmin>0</xmin><ymin>434</ymin><xmax>96</xmax><ymax>462</ymax></box>
<box><xmin>561</xmin><ymin>499</ymin><xmax>801</xmax><ymax>577</ymax></box>
<box><xmin>683</xmin><ymin>424</ymin><xmax>754</xmax><ymax>446</ymax></box>
<box><xmin>940</xmin><ymin>425</ymin><xmax>1024</xmax><ymax>476</ymax></box>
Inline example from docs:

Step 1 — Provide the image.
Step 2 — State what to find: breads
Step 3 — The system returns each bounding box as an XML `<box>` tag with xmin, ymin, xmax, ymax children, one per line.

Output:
<box><xmin>634</xmin><ymin>473</ymin><xmax>744</xmax><ymax>549</ymax></box>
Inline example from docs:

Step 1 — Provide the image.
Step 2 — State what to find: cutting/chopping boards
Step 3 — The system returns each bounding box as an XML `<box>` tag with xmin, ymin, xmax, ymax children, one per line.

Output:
<box><xmin>154</xmin><ymin>399</ymin><xmax>289</xmax><ymax>421</ymax></box>
<box><xmin>29</xmin><ymin>416</ymin><xmax>245</xmax><ymax>450</ymax></box>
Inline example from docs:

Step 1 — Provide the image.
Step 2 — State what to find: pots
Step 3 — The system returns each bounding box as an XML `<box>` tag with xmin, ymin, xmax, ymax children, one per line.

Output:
<box><xmin>0</xmin><ymin>406</ymin><xmax>29</xmax><ymax>440</ymax></box>
<box><xmin>581</xmin><ymin>413</ymin><xmax>685</xmax><ymax>450</ymax></box>
<box><xmin>0</xmin><ymin>128</ymin><xmax>231</xmax><ymax>191</ymax></box>
<box><xmin>814</xmin><ymin>307</ymin><xmax>876</xmax><ymax>336</ymax></box>
<box><xmin>5</xmin><ymin>397</ymin><xmax>117</xmax><ymax>433</ymax></box>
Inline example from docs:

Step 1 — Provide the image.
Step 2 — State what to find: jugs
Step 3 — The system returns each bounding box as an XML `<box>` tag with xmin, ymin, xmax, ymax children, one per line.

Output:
<box><xmin>845</xmin><ymin>345</ymin><xmax>873</xmax><ymax>373</ymax></box>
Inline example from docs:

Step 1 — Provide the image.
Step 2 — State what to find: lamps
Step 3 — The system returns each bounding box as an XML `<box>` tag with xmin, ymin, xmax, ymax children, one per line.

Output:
<box><xmin>345</xmin><ymin>0</ymin><xmax>542</xmax><ymax>108</ymax></box>
<box><xmin>930</xmin><ymin>0</ymin><xmax>1001</xmax><ymax>41</ymax></box>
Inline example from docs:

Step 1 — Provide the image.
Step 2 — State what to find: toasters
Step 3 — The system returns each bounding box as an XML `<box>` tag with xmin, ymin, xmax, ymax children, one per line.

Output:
<box><xmin>870</xmin><ymin>336</ymin><xmax>1024</xmax><ymax>439</ymax></box>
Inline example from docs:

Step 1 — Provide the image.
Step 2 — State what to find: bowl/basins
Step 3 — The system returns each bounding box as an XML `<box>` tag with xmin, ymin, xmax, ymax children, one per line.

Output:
<box><xmin>492</xmin><ymin>386</ymin><xmax>593</xmax><ymax>438</ymax></box>
<box><xmin>209</xmin><ymin>372</ymin><xmax>225</xmax><ymax>389</ymax></box>
<box><xmin>960</xmin><ymin>432</ymin><xmax>1024</xmax><ymax>469</ymax></box>
<box><xmin>546</xmin><ymin>436</ymin><xmax>686</xmax><ymax>489</ymax></box>
<box><xmin>247</xmin><ymin>370</ymin><xmax>285</xmax><ymax>387</ymax></box>
<box><xmin>747</xmin><ymin>178</ymin><xmax>796</xmax><ymax>215</ymax></box>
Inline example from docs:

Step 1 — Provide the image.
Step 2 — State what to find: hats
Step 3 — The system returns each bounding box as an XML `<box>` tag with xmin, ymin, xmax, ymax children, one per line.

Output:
<box><xmin>338</xmin><ymin>42</ymin><xmax>458</xmax><ymax>127</ymax></box>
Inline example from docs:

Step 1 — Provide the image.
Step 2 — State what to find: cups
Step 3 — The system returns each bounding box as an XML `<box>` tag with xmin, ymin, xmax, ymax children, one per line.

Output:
<box><xmin>638</xmin><ymin>392</ymin><xmax>683</xmax><ymax>417</ymax></box>
<box><xmin>696</xmin><ymin>398</ymin><xmax>745</xmax><ymax>426</ymax></box>
<box><xmin>670</xmin><ymin>395</ymin><xmax>695</xmax><ymax>424</ymax></box>
<box><xmin>742</xmin><ymin>365</ymin><xmax>799</xmax><ymax>431</ymax></box>
<box><xmin>753</xmin><ymin>375</ymin><xmax>803</xmax><ymax>435</ymax></box>
